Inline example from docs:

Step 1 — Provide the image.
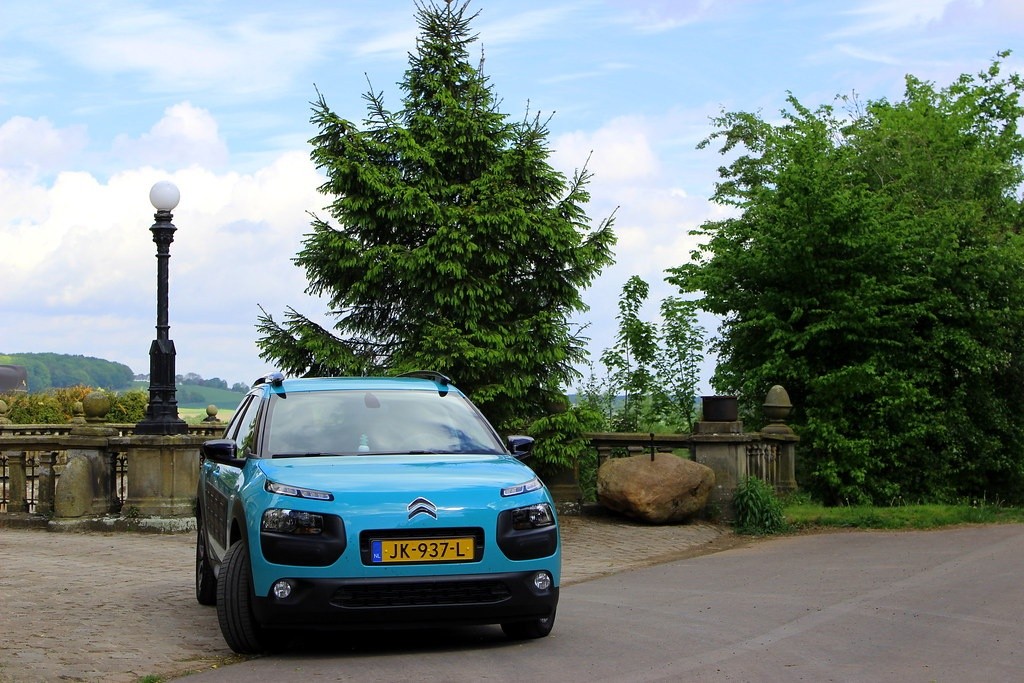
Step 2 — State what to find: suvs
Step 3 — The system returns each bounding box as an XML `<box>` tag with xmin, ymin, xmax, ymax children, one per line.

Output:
<box><xmin>196</xmin><ymin>371</ymin><xmax>562</xmax><ymax>656</ymax></box>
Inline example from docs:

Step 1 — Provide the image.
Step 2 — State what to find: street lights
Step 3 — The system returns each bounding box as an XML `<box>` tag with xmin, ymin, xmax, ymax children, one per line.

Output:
<box><xmin>133</xmin><ymin>180</ymin><xmax>190</xmax><ymax>433</ymax></box>
